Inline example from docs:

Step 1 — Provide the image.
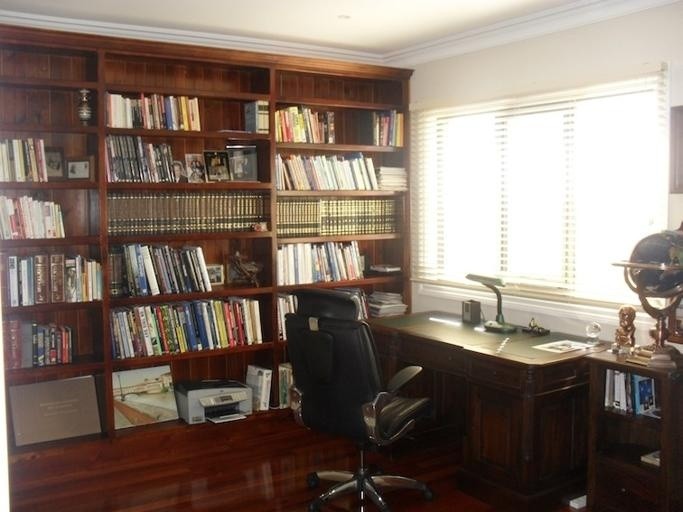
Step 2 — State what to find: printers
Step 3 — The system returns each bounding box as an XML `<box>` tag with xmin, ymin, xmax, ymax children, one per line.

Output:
<box><xmin>174</xmin><ymin>378</ymin><xmax>252</xmax><ymax>424</ymax></box>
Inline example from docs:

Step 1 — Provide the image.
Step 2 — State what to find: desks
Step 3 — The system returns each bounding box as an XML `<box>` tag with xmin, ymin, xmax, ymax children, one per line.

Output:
<box><xmin>370</xmin><ymin>311</ymin><xmax>616</xmax><ymax>499</ymax></box>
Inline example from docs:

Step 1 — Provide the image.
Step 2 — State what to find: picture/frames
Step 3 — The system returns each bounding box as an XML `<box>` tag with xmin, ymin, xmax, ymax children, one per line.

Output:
<box><xmin>184</xmin><ymin>146</ymin><xmax>259</xmax><ymax>183</ymax></box>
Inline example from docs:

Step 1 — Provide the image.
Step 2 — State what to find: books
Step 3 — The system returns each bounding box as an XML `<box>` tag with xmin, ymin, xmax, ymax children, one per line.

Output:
<box><xmin>640</xmin><ymin>450</ymin><xmax>661</xmax><ymax>467</ymax></box>
<box><xmin>2</xmin><ymin>252</ymin><xmax>102</xmax><ymax>307</ymax></box>
<box><xmin>1</xmin><ymin>195</ymin><xmax>65</xmax><ymax>240</ymax></box>
<box><xmin>107</xmin><ymin>243</ymin><xmax>212</xmax><ymax>296</ymax></box>
<box><xmin>108</xmin><ymin>296</ymin><xmax>263</xmax><ymax>359</ymax></box>
<box><xmin>107</xmin><ymin>191</ymin><xmax>264</xmax><ymax>233</ymax></box>
<box><xmin>7</xmin><ymin>320</ymin><xmax>74</xmax><ymax>370</ymax></box>
<box><xmin>1</xmin><ymin>138</ymin><xmax>47</xmax><ymax>182</ymax></box>
<box><xmin>274</xmin><ymin>101</ymin><xmax>409</xmax><ymax>339</ymax></box>
<box><xmin>531</xmin><ymin>339</ymin><xmax>595</xmax><ymax>354</ymax></box>
<box><xmin>107</xmin><ymin>95</ymin><xmax>201</xmax><ymax>132</ymax></box>
<box><xmin>604</xmin><ymin>369</ymin><xmax>655</xmax><ymax>414</ymax></box>
<box><xmin>106</xmin><ymin>134</ymin><xmax>257</xmax><ymax>183</ymax></box>
<box><xmin>244</xmin><ymin>365</ymin><xmax>293</xmax><ymax>412</ymax></box>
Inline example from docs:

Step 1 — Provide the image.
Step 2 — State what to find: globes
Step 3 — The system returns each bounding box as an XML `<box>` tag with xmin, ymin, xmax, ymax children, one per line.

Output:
<box><xmin>612</xmin><ymin>231</ymin><xmax>683</xmax><ymax>369</ymax></box>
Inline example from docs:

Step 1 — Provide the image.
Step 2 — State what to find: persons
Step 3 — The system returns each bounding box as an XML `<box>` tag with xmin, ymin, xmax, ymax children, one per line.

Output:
<box><xmin>47</xmin><ymin>149</ymin><xmax>61</xmax><ymax>174</ymax></box>
<box><xmin>614</xmin><ymin>307</ymin><xmax>634</xmax><ymax>347</ymax></box>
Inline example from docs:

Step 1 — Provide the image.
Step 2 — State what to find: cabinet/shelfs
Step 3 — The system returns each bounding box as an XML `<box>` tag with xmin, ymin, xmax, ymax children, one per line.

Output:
<box><xmin>584</xmin><ymin>352</ymin><xmax>682</xmax><ymax>511</ymax></box>
<box><xmin>0</xmin><ymin>21</ymin><xmax>413</xmax><ymax>454</ymax></box>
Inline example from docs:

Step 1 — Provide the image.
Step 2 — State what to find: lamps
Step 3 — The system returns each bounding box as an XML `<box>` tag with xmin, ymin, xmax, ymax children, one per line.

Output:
<box><xmin>464</xmin><ymin>273</ymin><xmax>518</xmax><ymax>333</ymax></box>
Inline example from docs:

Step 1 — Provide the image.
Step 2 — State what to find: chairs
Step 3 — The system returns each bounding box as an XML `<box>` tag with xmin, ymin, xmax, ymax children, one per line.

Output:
<box><xmin>277</xmin><ymin>283</ymin><xmax>440</xmax><ymax>512</ymax></box>
